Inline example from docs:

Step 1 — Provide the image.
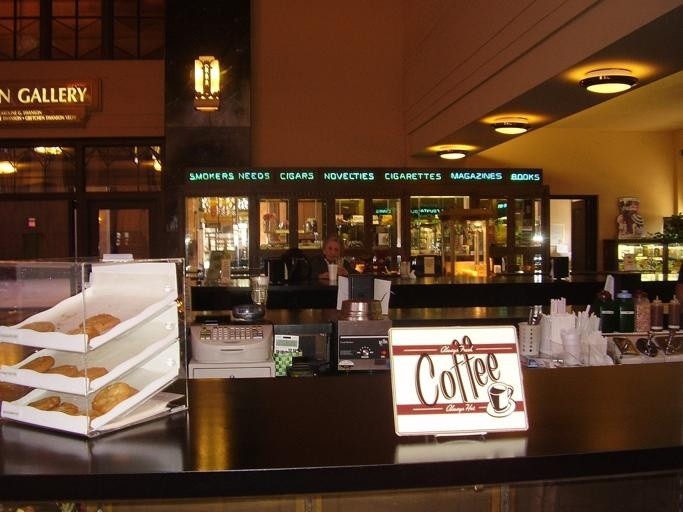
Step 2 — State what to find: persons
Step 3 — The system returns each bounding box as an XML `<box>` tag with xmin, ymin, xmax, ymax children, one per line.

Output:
<box><xmin>309</xmin><ymin>235</ymin><xmax>360</xmax><ymax>280</ymax></box>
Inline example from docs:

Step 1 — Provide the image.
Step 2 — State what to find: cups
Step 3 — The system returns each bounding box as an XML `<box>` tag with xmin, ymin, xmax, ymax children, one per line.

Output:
<box><xmin>327</xmin><ymin>264</ymin><xmax>338</xmax><ymax>280</ymax></box>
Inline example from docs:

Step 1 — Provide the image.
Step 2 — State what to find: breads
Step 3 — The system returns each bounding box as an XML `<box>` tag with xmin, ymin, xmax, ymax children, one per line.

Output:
<box><xmin>0</xmin><ymin>314</ymin><xmax>140</xmax><ymax>422</ymax></box>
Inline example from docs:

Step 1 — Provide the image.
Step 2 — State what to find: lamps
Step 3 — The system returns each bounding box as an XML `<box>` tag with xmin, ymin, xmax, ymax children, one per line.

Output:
<box><xmin>490</xmin><ymin>117</ymin><xmax>531</xmax><ymax>136</ymax></box>
<box><xmin>579</xmin><ymin>67</ymin><xmax>638</xmax><ymax>94</ymax></box>
<box><xmin>194</xmin><ymin>52</ymin><xmax>221</xmax><ymax>112</ymax></box>
<box><xmin>437</xmin><ymin>149</ymin><xmax>470</xmax><ymax>160</ymax></box>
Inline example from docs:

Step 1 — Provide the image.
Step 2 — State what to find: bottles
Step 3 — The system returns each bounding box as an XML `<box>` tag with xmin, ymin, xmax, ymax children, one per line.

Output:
<box><xmin>668</xmin><ymin>294</ymin><xmax>680</xmax><ymax>329</ymax></box>
<box><xmin>595</xmin><ymin>290</ymin><xmax>664</xmax><ymax>333</ymax></box>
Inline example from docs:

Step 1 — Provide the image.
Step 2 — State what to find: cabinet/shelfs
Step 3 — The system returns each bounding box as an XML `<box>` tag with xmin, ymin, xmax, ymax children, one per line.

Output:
<box><xmin>0</xmin><ymin>258</ymin><xmax>189</xmax><ymax>438</ymax></box>
